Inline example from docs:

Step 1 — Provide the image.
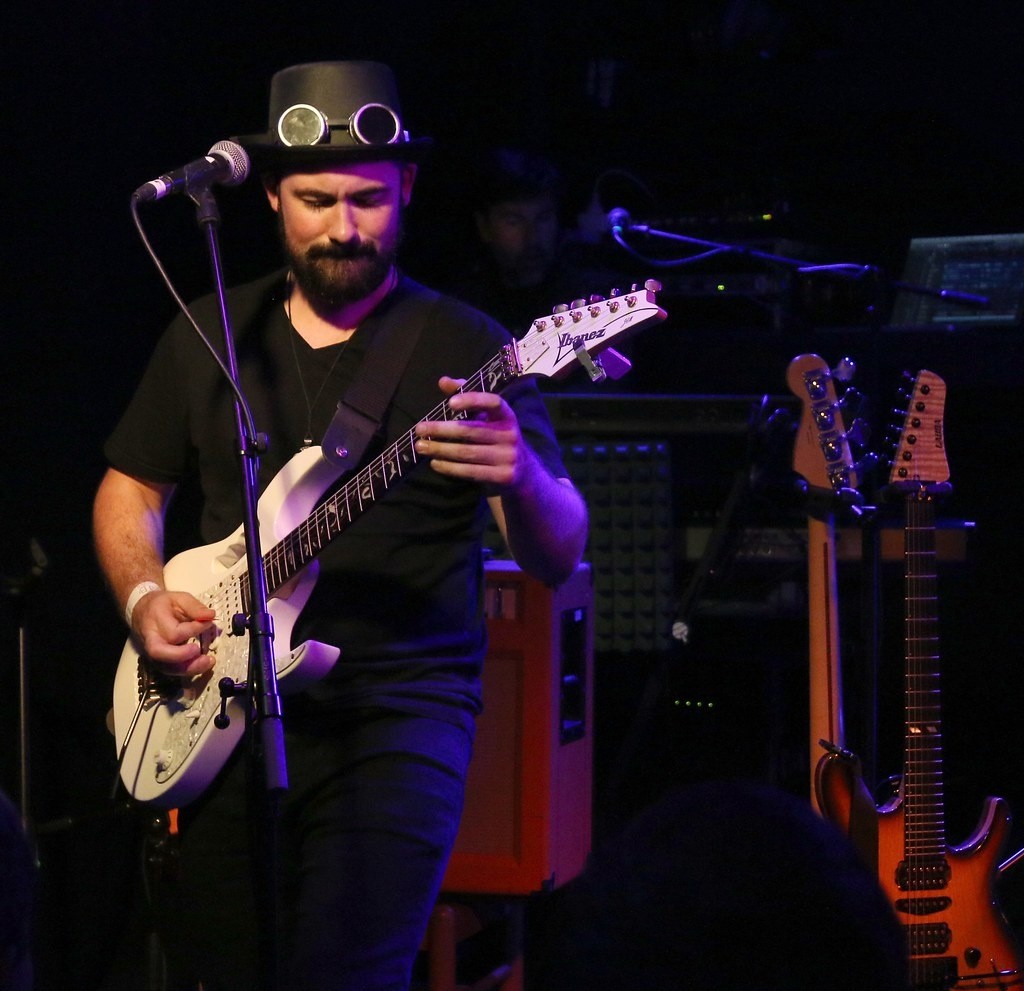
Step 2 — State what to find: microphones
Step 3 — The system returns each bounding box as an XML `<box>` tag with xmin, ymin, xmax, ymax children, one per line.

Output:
<box><xmin>132</xmin><ymin>140</ymin><xmax>251</xmax><ymax>204</ymax></box>
<box><xmin>582</xmin><ymin>192</ymin><xmax>606</xmax><ymax>245</ymax></box>
<box><xmin>609</xmin><ymin>208</ymin><xmax>631</xmax><ymax>235</ymax></box>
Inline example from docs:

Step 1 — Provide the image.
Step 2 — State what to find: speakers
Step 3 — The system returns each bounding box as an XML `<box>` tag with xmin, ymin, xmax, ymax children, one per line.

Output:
<box><xmin>437</xmin><ymin>558</ymin><xmax>593</xmax><ymax>896</ymax></box>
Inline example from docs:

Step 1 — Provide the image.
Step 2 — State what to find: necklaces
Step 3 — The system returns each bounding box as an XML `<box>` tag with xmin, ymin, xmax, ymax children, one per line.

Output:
<box><xmin>286</xmin><ymin>272</ymin><xmax>396</xmax><ymax>453</ymax></box>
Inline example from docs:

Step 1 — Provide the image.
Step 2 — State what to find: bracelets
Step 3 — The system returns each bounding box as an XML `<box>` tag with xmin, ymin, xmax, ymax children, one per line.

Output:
<box><xmin>124</xmin><ymin>581</ymin><xmax>162</xmax><ymax>628</ymax></box>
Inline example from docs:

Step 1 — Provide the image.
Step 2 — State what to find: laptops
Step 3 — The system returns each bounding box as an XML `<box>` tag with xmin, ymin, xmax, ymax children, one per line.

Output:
<box><xmin>889</xmin><ymin>232</ymin><xmax>1024</xmax><ymax>326</ymax></box>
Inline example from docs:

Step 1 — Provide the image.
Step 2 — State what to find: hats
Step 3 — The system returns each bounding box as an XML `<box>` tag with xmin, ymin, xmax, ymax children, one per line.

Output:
<box><xmin>228</xmin><ymin>60</ymin><xmax>434</xmax><ymax>162</ymax></box>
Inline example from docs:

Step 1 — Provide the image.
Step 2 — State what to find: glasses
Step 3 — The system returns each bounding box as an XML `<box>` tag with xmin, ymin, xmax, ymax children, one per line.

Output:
<box><xmin>278</xmin><ymin>102</ymin><xmax>402</xmax><ymax>146</ymax></box>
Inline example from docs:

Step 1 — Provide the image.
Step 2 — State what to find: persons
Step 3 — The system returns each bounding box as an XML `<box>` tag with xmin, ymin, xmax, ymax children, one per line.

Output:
<box><xmin>90</xmin><ymin>57</ymin><xmax>586</xmax><ymax>991</ymax></box>
<box><xmin>440</xmin><ymin>148</ymin><xmax>637</xmax><ymax>392</ymax></box>
<box><xmin>0</xmin><ymin>792</ymin><xmax>34</xmax><ymax>991</ymax></box>
<box><xmin>544</xmin><ymin>781</ymin><xmax>908</xmax><ymax>990</ymax></box>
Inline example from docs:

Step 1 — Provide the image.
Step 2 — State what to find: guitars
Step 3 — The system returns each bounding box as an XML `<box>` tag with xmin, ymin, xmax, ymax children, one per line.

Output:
<box><xmin>109</xmin><ymin>276</ymin><xmax>671</xmax><ymax>808</ymax></box>
<box><xmin>784</xmin><ymin>351</ymin><xmax>879</xmax><ymax>817</ymax></box>
<box><xmin>814</xmin><ymin>365</ymin><xmax>1024</xmax><ymax>990</ymax></box>
